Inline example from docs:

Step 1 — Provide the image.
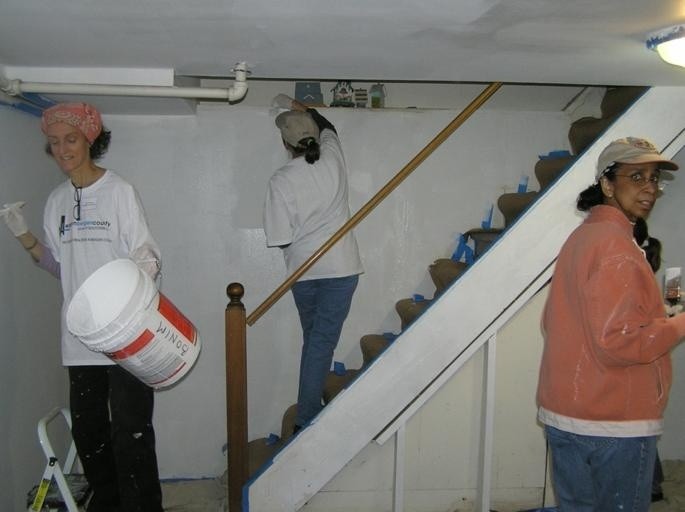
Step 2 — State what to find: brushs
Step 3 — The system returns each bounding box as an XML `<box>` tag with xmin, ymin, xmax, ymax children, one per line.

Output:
<box><xmin>664</xmin><ymin>266</ymin><xmax>681</xmax><ymax>306</ymax></box>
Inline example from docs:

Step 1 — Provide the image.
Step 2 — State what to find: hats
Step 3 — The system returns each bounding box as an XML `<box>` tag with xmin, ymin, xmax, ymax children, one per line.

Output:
<box><xmin>595</xmin><ymin>138</ymin><xmax>678</xmax><ymax>181</ymax></box>
<box><xmin>40</xmin><ymin>103</ymin><xmax>103</xmax><ymax>145</ymax></box>
<box><xmin>276</xmin><ymin>109</ymin><xmax>320</xmax><ymax>149</ymax></box>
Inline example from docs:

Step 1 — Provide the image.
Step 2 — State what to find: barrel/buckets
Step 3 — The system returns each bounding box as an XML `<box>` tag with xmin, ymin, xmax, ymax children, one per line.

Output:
<box><xmin>67</xmin><ymin>257</ymin><xmax>202</xmax><ymax>392</ymax></box>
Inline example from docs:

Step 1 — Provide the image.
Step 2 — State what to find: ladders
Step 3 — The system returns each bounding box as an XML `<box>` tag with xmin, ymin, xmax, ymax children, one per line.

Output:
<box><xmin>29</xmin><ymin>407</ymin><xmax>95</xmax><ymax>512</ymax></box>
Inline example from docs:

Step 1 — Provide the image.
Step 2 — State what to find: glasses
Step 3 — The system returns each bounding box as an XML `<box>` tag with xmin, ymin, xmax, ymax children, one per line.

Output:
<box><xmin>613</xmin><ymin>171</ymin><xmax>658</xmax><ymax>183</ymax></box>
<box><xmin>72</xmin><ymin>182</ymin><xmax>82</xmax><ymax>220</ymax></box>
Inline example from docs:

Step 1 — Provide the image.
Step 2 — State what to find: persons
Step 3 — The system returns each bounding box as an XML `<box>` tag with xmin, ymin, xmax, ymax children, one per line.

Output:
<box><xmin>1</xmin><ymin>100</ymin><xmax>163</xmax><ymax>512</ymax></box>
<box><xmin>630</xmin><ymin>216</ymin><xmax>665</xmax><ymax>503</ymax></box>
<box><xmin>265</xmin><ymin>93</ymin><xmax>367</xmax><ymax>435</ymax></box>
<box><xmin>536</xmin><ymin>137</ymin><xmax>684</xmax><ymax>512</ymax></box>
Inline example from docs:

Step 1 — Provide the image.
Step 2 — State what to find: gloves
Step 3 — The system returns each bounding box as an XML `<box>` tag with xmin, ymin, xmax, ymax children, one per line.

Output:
<box><xmin>135</xmin><ymin>248</ymin><xmax>159</xmax><ymax>278</ymax></box>
<box><xmin>2</xmin><ymin>203</ymin><xmax>29</xmax><ymax>238</ymax></box>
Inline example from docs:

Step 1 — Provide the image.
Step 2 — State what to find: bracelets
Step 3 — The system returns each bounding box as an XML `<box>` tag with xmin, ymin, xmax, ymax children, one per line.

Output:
<box><xmin>25</xmin><ymin>236</ymin><xmax>38</xmax><ymax>250</ymax></box>
